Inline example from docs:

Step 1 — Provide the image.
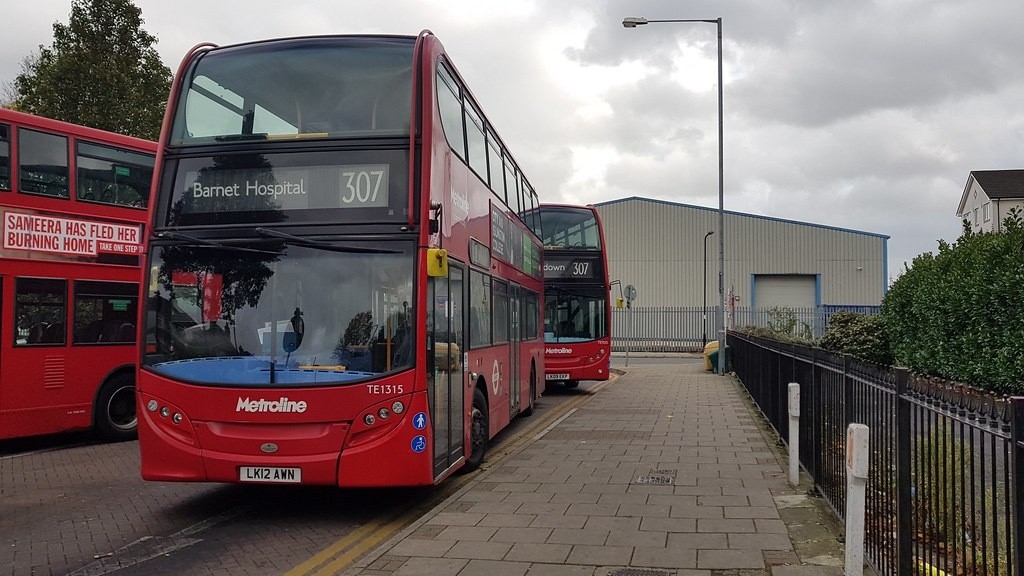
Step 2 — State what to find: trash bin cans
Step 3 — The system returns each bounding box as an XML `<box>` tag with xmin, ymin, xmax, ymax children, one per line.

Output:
<box><xmin>704</xmin><ymin>341</ymin><xmax>719</xmax><ymax>371</ymax></box>
<box><xmin>709</xmin><ymin>345</ymin><xmax>732</xmax><ymax>374</ymax></box>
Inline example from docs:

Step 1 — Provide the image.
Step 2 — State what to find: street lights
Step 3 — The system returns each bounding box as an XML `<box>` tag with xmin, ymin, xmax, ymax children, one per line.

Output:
<box><xmin>620</xmin><ymin>16</ymin><xmax>727</xmax><ymax>376</ymax></box>
<box><xmin>702</xmin><ymin>230</ymin><xmax>714</xmax><ymax>349</ymax></box>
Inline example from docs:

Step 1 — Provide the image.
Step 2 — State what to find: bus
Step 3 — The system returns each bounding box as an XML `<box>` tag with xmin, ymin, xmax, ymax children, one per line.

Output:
<box><xmin>134</xmin><ymin>27</ymin><xmax>546</xmax><ymax>489</ymax></box>
<box><xmin>0</xmin><ymin>107</ymin><xmax>221</xmax><ymax>440</ymax></box>
<box><xmin>538</xmin><ymin>200</ymin><xmax>624</xmax><ymax>389</ymax></box>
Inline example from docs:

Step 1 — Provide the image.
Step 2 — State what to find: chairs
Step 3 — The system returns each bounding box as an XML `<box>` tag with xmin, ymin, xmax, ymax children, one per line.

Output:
<box><xmin>25</xmin><ymin>319</ymin><xmax>137</xmax><ymax>343</ymax></box>
<box><xmin>556</xmin><ymin>320</ymin><xmax>576</xmax><ymax>337</ymax></box>
<box><xmin>20</xmin><ymin>177</ymin><xmax>113</xmax><ymax>203</ymax></box>
